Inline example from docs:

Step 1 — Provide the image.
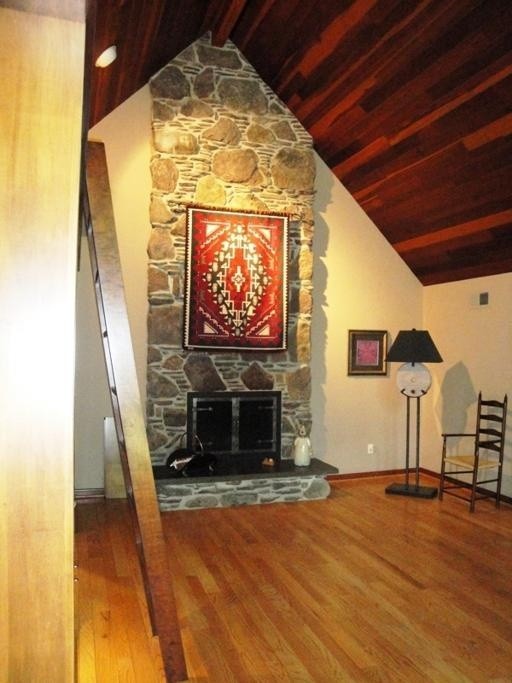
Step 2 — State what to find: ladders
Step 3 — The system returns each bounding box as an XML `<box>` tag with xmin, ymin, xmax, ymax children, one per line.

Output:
<box><xmin>81</xmin><ymin>141</ymin><xmax>188</xmax><ymax>683</ymax></box>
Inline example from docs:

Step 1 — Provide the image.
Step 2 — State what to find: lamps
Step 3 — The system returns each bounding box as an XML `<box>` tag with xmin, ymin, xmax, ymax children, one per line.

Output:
<box><xmin>384</xmin><ymin>328</ymin><xmax>443</xmax><ymax>497</ymax></box>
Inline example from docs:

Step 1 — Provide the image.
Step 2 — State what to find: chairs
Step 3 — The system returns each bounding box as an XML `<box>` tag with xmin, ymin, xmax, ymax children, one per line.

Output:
<box><xmin>439</xmin><ymin>390</ymin><xmax>508</xmax><ymax>513</ymax></box>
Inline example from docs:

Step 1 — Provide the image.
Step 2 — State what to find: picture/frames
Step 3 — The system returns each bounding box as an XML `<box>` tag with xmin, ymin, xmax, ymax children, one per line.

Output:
<box><xmin>347</xmin><ymin>329</ymin><xmax>388</xmax><ymax>375</ymax></box>
